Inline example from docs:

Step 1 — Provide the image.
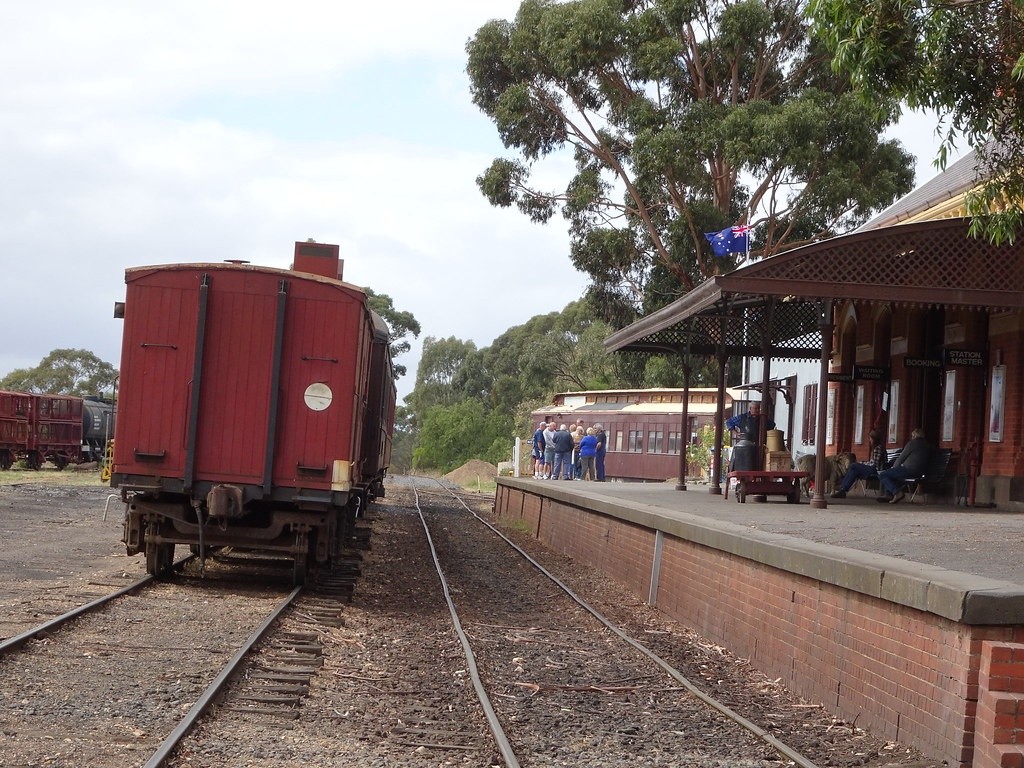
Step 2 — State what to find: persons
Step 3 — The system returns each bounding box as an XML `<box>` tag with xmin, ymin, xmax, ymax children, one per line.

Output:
<box><xmin>532</xmin><ymin>418</ymin><xmax>607</xmax><ymax>481</ymax></box>
<box><xmin>726</xmin><ymin>402</ymin><xmax>776</xmax><ymax>443</ymax></box>
<box><xmin>880</xmin><ymin>428</ymin><xmax>931</xmax><ymax>503</ymax></box>
<box><xmin>830</xmin><ymin>430</ymin><xmax>887</xmax><ymax>499</ymax></box>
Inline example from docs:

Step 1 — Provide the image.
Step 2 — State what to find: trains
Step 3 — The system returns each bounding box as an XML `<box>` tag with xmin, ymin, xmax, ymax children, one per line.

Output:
<box><xmin>0</xmin><ymin>385</ymin><xmax>121</xmax><ymax>470</ymax></box>
<box><xmin>99</xmin><ymin>236</ymin><xmax>395</xmax><ymax>593</ymax></box>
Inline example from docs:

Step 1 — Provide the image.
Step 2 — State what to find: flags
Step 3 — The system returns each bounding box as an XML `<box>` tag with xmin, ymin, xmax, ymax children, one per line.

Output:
<box><xmin>704</xmin><ymin>225</ymin><xmax>751</xmax><ymax>256</ymax></box>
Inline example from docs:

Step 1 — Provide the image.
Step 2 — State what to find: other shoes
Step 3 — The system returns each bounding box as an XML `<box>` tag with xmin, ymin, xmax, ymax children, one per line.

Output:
<box><xmin>533</xmin><ymin>475</ymin><xmax>584</xmax><ymax>481</ymax></box>
<box><xmin>890</xmin><ymin>491</ymin><xmax>906</xmax><ymax>504</ymax></box>
<box><xmin>594</xmin><ymin>478</ymin><xmax>606</xmax><ymax>482</ymax></box>
<box><xmin>877</xmin><ymin>495</ymin><xmax>894</xmax><ymax>502</ymax></box>
<box><xmin>831</xmin><ymin>491</ymin><xmax>846</xmax><ymax>498</ymax></box>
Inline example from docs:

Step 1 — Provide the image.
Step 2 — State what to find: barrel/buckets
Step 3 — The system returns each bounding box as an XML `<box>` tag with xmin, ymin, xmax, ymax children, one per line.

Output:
<box><xmin>766</xmin><ymin>427</ymin><xmax>785</xmax><ymax>451</ymax></box>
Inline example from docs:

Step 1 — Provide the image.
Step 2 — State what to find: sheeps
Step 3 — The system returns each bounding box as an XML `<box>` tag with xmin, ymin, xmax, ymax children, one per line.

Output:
<box><xmin>796</xmin><ymin>452</ymin><xmax>857</xmax><ymax>498</ymax></box>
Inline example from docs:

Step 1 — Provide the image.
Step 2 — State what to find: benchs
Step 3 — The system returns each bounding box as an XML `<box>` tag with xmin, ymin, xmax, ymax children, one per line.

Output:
<box><xmin>861</xmin><ymin>447</ymin><xmax>952</xmax><ymax>503</ymax></box>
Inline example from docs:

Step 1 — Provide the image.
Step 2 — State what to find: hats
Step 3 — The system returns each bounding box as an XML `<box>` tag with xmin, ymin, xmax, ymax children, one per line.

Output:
<box><xmin>539</xmin><ymin>421</ymin><xmax>549</xmax><ymax>426</ymax></box>
<box><xmin>867</xmin><ymin>430</ymin><xmax>881</xmax><ymax>441</ymax></box>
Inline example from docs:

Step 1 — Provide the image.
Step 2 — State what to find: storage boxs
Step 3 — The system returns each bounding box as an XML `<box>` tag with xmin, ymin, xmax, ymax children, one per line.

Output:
<box><xmin>766</xmin><ymin>450</ymin><xmax>790</xmax><ymax>472</ymax></box>
